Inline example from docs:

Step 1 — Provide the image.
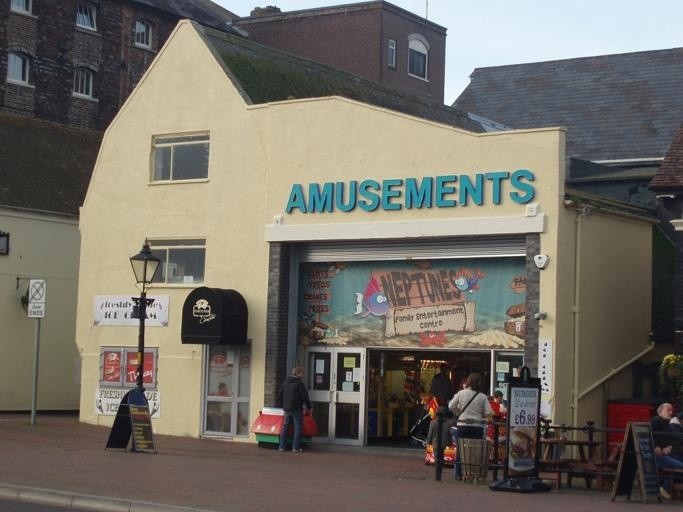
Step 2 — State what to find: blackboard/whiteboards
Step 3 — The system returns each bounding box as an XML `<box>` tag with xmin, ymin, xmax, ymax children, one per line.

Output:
<box><xmin>631</xmin><ymin>422</ymin><xmax>660</xmax><ymax>497</ymax></box>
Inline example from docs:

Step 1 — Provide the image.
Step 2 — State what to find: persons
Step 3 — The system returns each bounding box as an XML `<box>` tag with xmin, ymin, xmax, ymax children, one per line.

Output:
<box><xmin>494</xmin><ymin>391</ymin><xmax>507</xmax><ymax>415</ymax></box>
<box><xmin>668</xmin><ymin>410</ymin><xmax>683</xmax><ymax>443</ymax></box>
<box><xmin>277</xmin><ymin>368</ymin><xmax>311</xmax><ymax>453</ymax></box>
<box><xmin>427</xmin><ymin>407</ymin><xmax>453</xmax><ymax>472</ymax></box>
<box><xmin>449</xmin><ymin>373</ymin><xmax>494</xmax><ymax>480</ymax></box>
<box><xmin>487</xmin><ymin>395</ymin><xmax>499</xmax><ymax>414</ymax></box>
<box><xmin>649</xmin><ymin>403</ymin><xmax>682</xmax><ymax>500</ymax></box>
<box><xmin>431</xmin><ymin>364</ymin><xmax>453</xmax><ymax>405</ymax></box>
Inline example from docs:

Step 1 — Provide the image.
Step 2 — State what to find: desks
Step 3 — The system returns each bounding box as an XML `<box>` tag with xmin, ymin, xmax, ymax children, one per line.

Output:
<box><xmin>540</xmin><ymin>437</ymin><xmax>598</xmax><ymax>491</ymax></box>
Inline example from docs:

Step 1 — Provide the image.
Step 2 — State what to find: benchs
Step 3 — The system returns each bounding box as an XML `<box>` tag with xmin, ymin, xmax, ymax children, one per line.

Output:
<box><xmin>487</xmin><ymin>461</ymin><xmax>565</xmax><ymax>491</ymax></box>
<box><xmin>663</xmin><ymin>467</ymin><xmax>683</xmax><ymax>500</ymax></box>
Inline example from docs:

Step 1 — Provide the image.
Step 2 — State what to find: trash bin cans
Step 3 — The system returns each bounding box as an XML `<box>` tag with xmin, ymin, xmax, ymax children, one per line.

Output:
<box><xmin>459</xmin><ymin>437</ymin><xmax>488</xmax><ymax>484</ymax></box>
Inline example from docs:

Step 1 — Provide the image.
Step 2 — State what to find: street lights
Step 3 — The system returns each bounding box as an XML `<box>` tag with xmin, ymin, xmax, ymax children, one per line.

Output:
<box><xmin>127</xmin><ymin>237</ymin><xmax>160</xmax><ymax>454</ymax></box>
